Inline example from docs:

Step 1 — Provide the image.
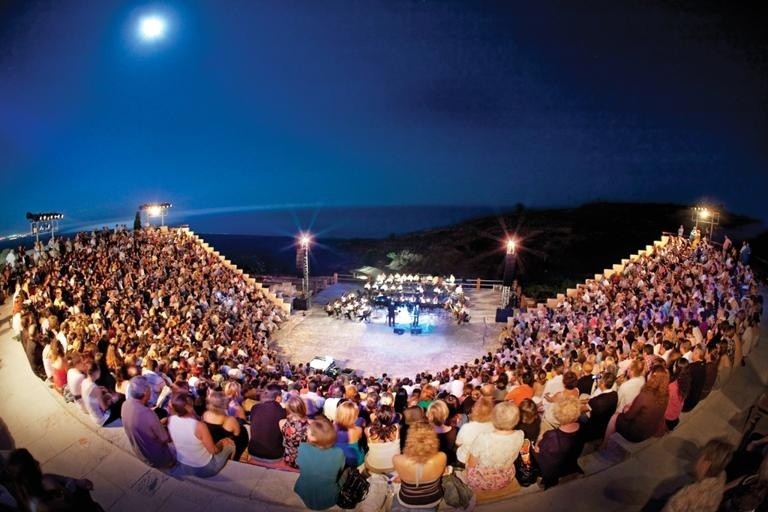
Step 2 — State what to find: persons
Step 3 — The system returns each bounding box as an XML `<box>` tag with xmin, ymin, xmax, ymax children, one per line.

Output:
<box><xmin>1</xmin><ymin>222</ymin><xmax>285</xmax><ymax>512</ymax></box>
<box><xmin>323</xmin><ymin>272</ymin><xmax>469</xmax><ymax>329</ymax></box>
<box><xmin>285</xmin><ymin>363</ymin><xmax>768</xmax><ymax>512</ymax></box>
<box><xmin>472</xmin><ymin>223</ymin><xmax>759</xmax><ymax>363</ymax></box>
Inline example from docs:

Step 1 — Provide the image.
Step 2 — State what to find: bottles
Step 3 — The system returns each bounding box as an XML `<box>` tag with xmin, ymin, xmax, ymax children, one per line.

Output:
<box><xmin>385</xmin><ymin>476</ymin><xmax>394</xmax><ymax>496</ymax></box>
<box><xmin>522</xmin><ymin>447</ymin><xmax>531</xmax><ymax>470</ymax></box>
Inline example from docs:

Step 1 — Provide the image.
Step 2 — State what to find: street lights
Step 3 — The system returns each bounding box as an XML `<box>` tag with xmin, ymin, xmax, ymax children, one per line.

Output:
<box><xmin>27</xmin><ymin>212</ymin><xmax>64</xmax><ymax>249</ymax></box>
<box><xmin>692</xmin><ymin>204</ymin><xmax>720</xmax><ymax>244</ymax></box>
<box><xmin>139</xmin><ymin>203</ymin><xmax>174</xmax><ymax>227</ymax></box>
<box><xmin>504</xmin><ymin>236</ymin><xmax>516</xmax><ymax>286</ymax></box>
<box><xmin>298</xmin><ymin>230</ymin><xmax>312</xmax><ymax>308</ymax></box>
